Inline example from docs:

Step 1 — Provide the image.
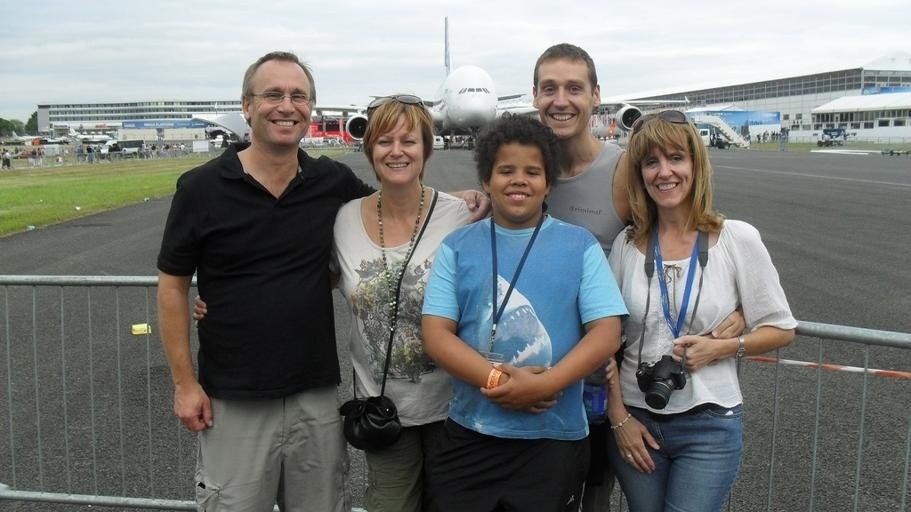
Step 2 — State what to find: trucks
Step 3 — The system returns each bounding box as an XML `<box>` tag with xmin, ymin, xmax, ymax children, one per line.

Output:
<box><xmin>698</xmin><ymin>129</ymin><xmax>741</xmax><ymax>150</ymax></box>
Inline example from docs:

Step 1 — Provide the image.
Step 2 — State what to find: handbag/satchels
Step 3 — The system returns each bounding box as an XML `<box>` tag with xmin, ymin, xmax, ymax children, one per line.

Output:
<box><xmin>337</xmin><ymin>395</ymin><xmax>400</xmax><ymax>450</ymax></box>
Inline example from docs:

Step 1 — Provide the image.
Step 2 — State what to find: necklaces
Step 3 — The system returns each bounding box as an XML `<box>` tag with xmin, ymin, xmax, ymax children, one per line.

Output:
<box><xmin>374</xmin><ymin>186</ymin><xmax>425</xmax><ymax>330</ymax></box>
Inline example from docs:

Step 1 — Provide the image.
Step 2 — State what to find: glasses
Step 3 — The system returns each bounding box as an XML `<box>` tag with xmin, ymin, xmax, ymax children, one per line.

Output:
<box><xmin>367</xmin><ymin>95</ymin><xmax>425</xmax><ymax>116</ymax></box>
<box><xmin>245</xmin><ymin>90</ymin><xmax>313</xmax><ymax>107</ymax></box>
<box><xmin>630</xmin><ymin>110</ymin><xmax>689</xmax><ymax>142</ymax></box>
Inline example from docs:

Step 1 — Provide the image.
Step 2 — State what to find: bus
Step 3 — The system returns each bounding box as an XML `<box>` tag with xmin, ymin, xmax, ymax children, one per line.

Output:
<box><xmin>101</xmin><ymin>139</ymin><xmax>146</xmax><ymax>159</ymax></box>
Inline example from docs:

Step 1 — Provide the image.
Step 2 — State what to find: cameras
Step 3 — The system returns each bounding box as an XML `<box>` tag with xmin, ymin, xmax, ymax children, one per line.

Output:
<box><xmin>635</xmin><ymin>355</ymin><xmax>687</xmax><ymax>410</ymax></box>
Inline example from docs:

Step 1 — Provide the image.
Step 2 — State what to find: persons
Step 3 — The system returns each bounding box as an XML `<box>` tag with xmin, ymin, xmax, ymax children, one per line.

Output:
<box><xmin>191</xmin><ymin>94</ymin><xmax>485</xmax><ymax>512</ymax></box>
<box><xmin>526</xmin><ymin>39</ymin><xmax>752</xmax><ymax>512</ymax></box>
<box><xmin>419</xmin><ymin>109</ymin><xmax>634</xmax><ymax>512</ymax></box>
<box><xmin>595</xmin><ymin>107</ymin><xmax>799</xmax><ymax>512</ymax></box>
<box><xmin>153</xmin><ymin>49</ymin><xmax>493</xmax><ymax>512</ymax></box>
<box><xmin>708</xmin><ymin>123</ymin><xmax>793</xmax><ymax>151</ymax></box>
<box><xmin>0</xmin><ymin>133</ymin><xmax>189</xmax><ymax>169</ymax></box>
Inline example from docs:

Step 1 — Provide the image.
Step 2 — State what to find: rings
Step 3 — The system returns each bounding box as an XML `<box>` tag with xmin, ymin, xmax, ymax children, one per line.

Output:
<box><xmin>624</xmin><ymin>454</ymin><xmax>632</xmax><ymax>461</ymax></box>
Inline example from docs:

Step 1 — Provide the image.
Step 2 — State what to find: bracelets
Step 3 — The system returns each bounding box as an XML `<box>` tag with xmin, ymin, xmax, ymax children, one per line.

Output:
<box><xmin>734</xmin><ymin>334</ymin><xmax>746</xmax><ymax>364</ymax></box>
<box><xmin>609</xmin><ymin>413</ymin><xmax>632</xmax><ymax>434</ymax></box>
<box><xmin>485</xmin><ymin>367</ymin><xmax>502</xmax><ymax>391</ymax></box>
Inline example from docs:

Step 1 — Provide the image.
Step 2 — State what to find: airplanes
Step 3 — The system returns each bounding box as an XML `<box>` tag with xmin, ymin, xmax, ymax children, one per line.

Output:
<box><xmin>11</xmin><ymin>128</ymin><xmax>113</xmax><ymax>144</ymax></box>
<box><xmin>313</xmin><ymin>18</ymin><xmax>689</xmax><ymax>149</ymax></box>
